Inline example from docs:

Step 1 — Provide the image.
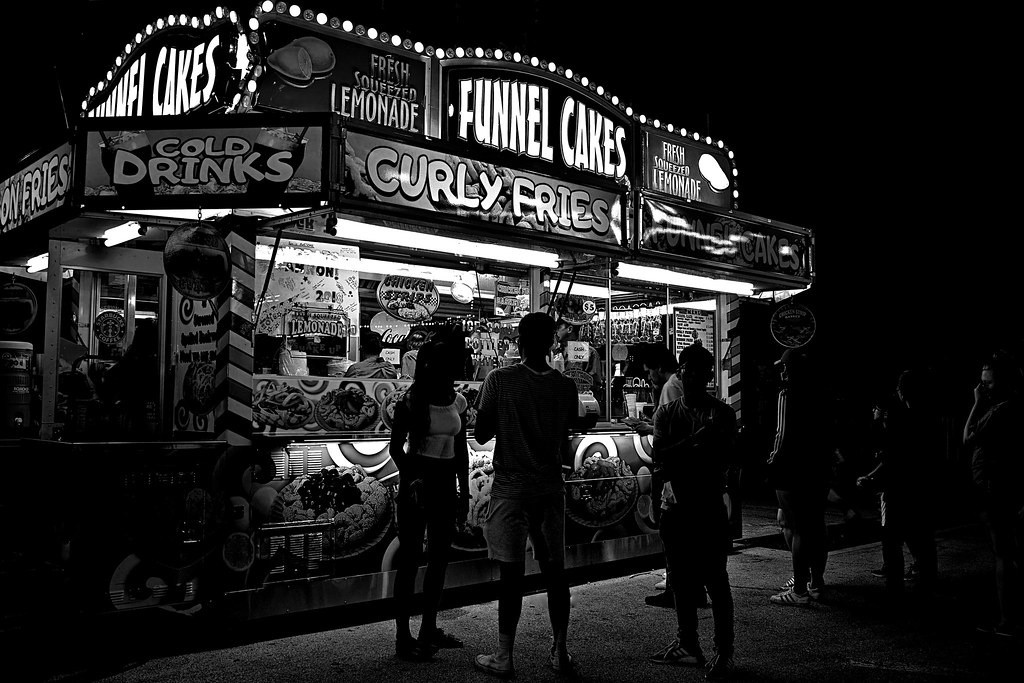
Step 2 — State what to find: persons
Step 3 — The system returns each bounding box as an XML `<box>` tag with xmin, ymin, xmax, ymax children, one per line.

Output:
<box><xmin>389</xmin><ymin>340</ymin><xmax>470</xmax><ymax>659</ymax></box>
<box><xmin>474</xmin><ymin>313</ymin><xmax>579</xmax><ymax>676</ymax></box>
<box><xmin>630</xmin><ymin>340</ymin><xmax>1024</xmax><ymax>680</ymax></box>
<box><xmin>344</xmin><ymin>332</ymin><xmax>428</xmax><ymax>379</ymax></box>
<box><xmin>556</xmin><ymin>319</ymin><xmax>601</xmax><ymax>391</ymax></box>
<box><xmin>102</xmin><ymin>318</ymin><xmax>160</xmax><ymax>405</ymax></box>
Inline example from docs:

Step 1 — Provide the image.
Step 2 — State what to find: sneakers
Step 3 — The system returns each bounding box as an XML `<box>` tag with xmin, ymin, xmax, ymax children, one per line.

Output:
<box><xmin>704</xmin><ymin>652</ymin><xmax>736</xmax><ymax>680</ymax></box>
<box><xmin>770</xmin><ymin>586</ymin><xmax>810</xmax><ymax>604</ymax></box>
<box><xmin>648</xmin><ymin>637</ymin><xmax>705</xmax><ymax>664</ymax></box>
<box><xmin>806</xmin><ymin>582</ymin><xmax>826</xmax><ymax>600</ymax></box>
<box><xmin>551</xmin><ymin>651</ymin><xmax>572</xmax><ymax>671</ymax></box>
<box><xmin>475</xmin><ymin>653</ymin><xmax>511</xmax><ymax>675</ymax></box>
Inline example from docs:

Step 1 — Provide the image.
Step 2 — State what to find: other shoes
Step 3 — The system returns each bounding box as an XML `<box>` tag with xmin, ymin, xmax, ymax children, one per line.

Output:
<box><xmin>871</xmin><ymin>564</ymin><xmax>889</xmax><ymax>578</ymax></box>
<box><xmin>394</xmin><ymin>637</ymin><xmax>440</xmax><ymax>659</ymax></box>
<box><xmin>418</xmin><ymin>628</ymin><xmax>464</xmax><ymax>648</ymax></box>
<box><xmin>694</xmin><ymin>588</ymin><xmax>710</xmax><ymax>607</ymax></box>
<box><xmin>904</xmin><ymin>563</ymin><xmax>937</xmax><ymax>582</ymax></box>
<box><xmin>644</xmin><ymin>591</ymin><xmax>675</xmax><ymax>609</ymax></box>
